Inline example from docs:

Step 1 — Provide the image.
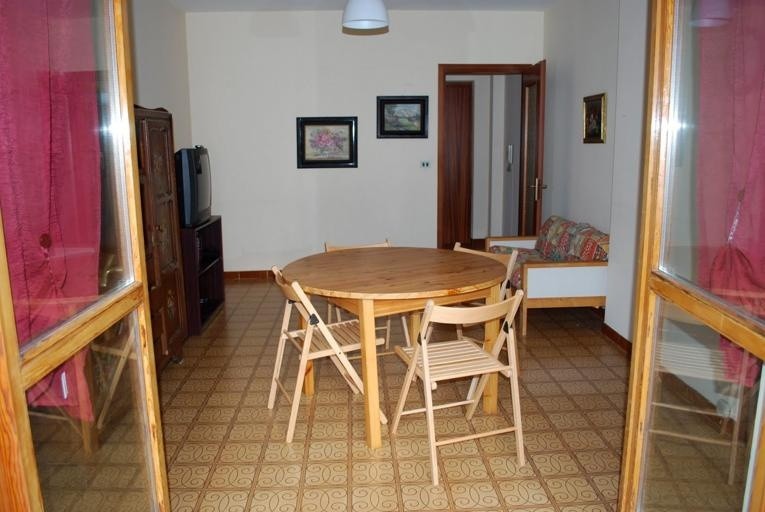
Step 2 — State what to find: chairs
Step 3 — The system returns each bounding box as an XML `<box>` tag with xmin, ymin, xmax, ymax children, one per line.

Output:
<box><xmin>88</xmin><ymin>315</ymin><xmax>140</xmax><ymax>432</ymax></box>
<box><xmin>26</xmin><ymin>375</ymin><xmax>83</xmax><ymax>444</ymax></box>
<box><xmin>387</xmin><ymin>288</ymin><xmax>529</xmax><ymax>486</ymax></box>
<box><xmin>266</xmin><ymin>265</ymin><xmax>387</xmax><ymax>444</ymax></box>
<box><xmin>320</xmin><ymin>236</ymin><xmax>416</xmax><ymax>384</ymax></box>
<box><xmin>408</xmin><ymin>237</ymin><xmax>522</xmax><ymax>400</ymax></box>
<box><xmin>648</xmin><ymin>285</ymin><xmax>754</xmax><ymax>492</ymax></box>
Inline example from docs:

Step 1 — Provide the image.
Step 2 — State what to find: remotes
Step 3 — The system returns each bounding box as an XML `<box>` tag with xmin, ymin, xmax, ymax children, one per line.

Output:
<box><xmin>196</xmin><ymin>145</ymin><xmax>203</xmax><ymax>148</ymax></box>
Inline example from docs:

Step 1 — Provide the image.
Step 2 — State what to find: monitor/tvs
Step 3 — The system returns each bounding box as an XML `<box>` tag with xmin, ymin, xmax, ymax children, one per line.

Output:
<box><xmin>174</xmin><ymin>149</ymin><xmax>212</xmax><ymax>227</ymax></box>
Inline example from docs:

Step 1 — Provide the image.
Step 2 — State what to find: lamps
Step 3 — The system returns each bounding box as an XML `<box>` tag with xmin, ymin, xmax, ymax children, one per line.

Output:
<box><xmin>340</xmin><ymin>0</ymin><xmax>390</xmax><ymax>33</ymax></box>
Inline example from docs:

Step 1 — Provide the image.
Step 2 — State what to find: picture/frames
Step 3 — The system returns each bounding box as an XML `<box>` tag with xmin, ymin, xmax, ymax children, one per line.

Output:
<box><xmin>295</xmin><ymin>115</ymin><xmax>360</xmax><ymax>171</ymax></box>
<box><xmin>581</xmin><ymin>92</ymin><xmax>608</xmax><ymax>146</ymax></box>
<box><xmin>375</xmin><ymin>94</ymin><xmax>429</xmax><ymax>140</ymax></box>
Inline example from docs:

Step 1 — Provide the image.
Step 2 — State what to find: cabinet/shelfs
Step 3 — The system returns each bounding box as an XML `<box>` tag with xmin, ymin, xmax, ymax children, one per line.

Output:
<box><xmin>100</xmin><ymin>105</ymin><xmax>190</xmax><ymax>383</ymax></box>
<box><xmin>179</xmin><ymin>215</ymin><xmax>229</xmax><ymax>338</ymax></box>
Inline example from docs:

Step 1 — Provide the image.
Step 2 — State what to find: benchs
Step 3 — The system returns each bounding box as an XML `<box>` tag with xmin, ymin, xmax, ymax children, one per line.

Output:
<box><xmin>482</xmin><ymin>213</ymin><xmax>611</xmax><ymax>342</ymax></box>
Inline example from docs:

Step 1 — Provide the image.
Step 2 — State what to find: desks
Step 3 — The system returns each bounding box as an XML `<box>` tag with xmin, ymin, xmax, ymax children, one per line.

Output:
<box><xmin>2</xmin><ymin>268</ymin><xmax>133</xmax><ymax>457</ymax></box>
<box><xmin>280</xmin><ymin>244</ymin><xmax>507</xmax><ymax>449</ymax></box>
